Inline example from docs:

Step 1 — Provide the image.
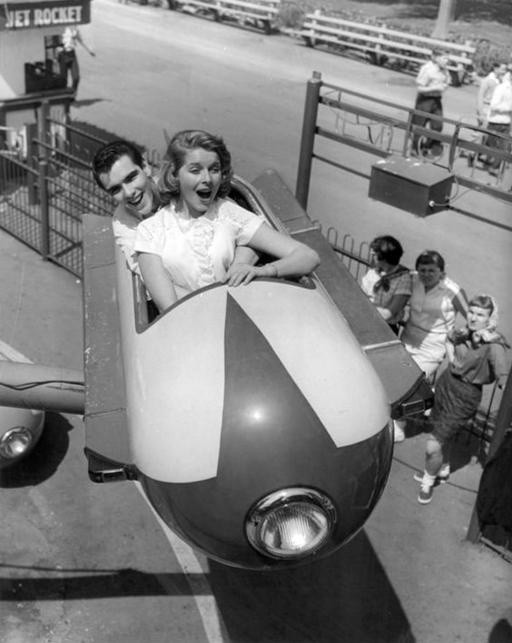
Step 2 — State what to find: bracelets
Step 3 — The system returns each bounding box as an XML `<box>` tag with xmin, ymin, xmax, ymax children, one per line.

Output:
<box><xmin>265</xmin><ymin>262</ymin><xmax>278</xmax><ymax>277</ymax></box>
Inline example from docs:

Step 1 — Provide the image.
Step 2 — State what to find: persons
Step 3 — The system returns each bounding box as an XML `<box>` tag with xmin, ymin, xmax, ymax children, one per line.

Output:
<box><xmin>58</xmin><ymin>26</ymin><xmax>95</xmax><ymax>102</ymax></box>
<box><xmin>130</xmin><ymin>126</ymin><xmax>322</xmax><ymax>315</ymax></box>
<box><xmin>91</xmin><ymin>139</ymin><xmax>260</xmax><ymax>325</ymax></box>
<box><xmin>390</xmin><ymin>248</ymin><xmax>470</xmax><ymax>442</ymax></box>
<box><xmin>410</xmin><ymin>46</ymin><xmax>455</xmax><ymax>157</ymax></box>
<box><xmin>413</xmin><ymin>292</ymin><xmax>509</xmax><ymax>506</ymax></box>
<box><xmin>359</xmin><ymin>233</ymin><xmax>412</xmax><ymax>337</ymax></box>
<box><xmin>462</xmin><ymin>60</ymin><xmax>512</xmax><ymax>178</ymax></box>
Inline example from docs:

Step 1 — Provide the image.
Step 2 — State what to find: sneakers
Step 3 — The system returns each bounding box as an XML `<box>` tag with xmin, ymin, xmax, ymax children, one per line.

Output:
<box><xmin>393</xmin><ymin>430</ymin><xmax>405</xmax><ymax>443</ymax></box>
<box><xmin>413</xmin><ymin>470</ymin><xmax>450</xmax><ymax>484</ymax></box>
<box><xmin>418</xmin><ymin>483</ymin><xmax>433</xmax><ymax>503</ymax></box>
